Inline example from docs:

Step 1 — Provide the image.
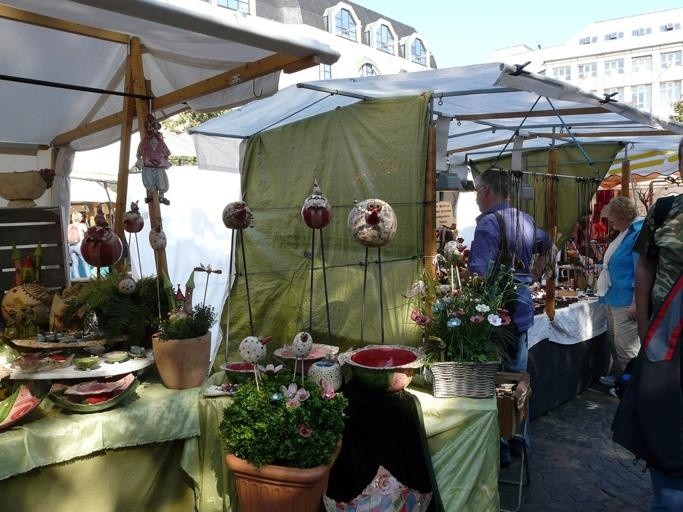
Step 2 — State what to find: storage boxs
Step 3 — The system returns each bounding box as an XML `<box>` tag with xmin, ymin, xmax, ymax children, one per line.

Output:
<box><xmin>495</xmin><ymin>369</ymin><xmax>535</xmax><ymax>441</ymax></box>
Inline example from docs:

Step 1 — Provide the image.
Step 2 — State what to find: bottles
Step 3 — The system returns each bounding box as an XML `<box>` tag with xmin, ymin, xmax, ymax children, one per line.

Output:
<box><xmin>307</xmin><ymin>360</ymin><xmax>342</xmax><ymax>392</ymax></box>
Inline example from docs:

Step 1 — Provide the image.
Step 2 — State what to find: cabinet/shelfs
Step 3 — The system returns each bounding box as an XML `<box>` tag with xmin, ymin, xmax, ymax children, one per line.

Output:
<box><xmin>0</xmin><ymin>203</ymin><xmax>70</xmax><ymax>303</ymax></box>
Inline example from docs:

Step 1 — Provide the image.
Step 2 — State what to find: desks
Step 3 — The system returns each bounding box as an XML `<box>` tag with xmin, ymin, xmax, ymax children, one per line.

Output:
<box><xmin>522</xmin><ymin>293</ymin><xmax>613</xmax><ymax>422</ymax></box>
<box><xmin>201</xmin><ymin>377</ymin><xmax>505</xmax><ymax>511</ymax></box>
<box><xmin>0</xmin><ymin>367</ymin><xmax>224</xmax><ymax>512</ymax></box>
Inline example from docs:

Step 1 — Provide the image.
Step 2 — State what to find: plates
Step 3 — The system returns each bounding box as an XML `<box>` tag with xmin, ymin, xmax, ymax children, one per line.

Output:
<box><xmin>0</xmin><ymin>345</ymin><xmax>147</xmax><ymax>428</ymax></box>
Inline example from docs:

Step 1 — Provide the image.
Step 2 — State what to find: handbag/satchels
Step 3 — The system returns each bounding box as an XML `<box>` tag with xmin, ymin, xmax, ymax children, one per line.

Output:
<box><xmin>493</xmin><ymin>273</ymin><xmax>521</xmax><ymax>321</ymax></box>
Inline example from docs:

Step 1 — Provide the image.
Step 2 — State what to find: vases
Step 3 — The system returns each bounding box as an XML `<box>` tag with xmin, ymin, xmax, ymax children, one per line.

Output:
<box><xmin>221</xmin><ymin>444</ymin><xmax>343</xmax><ymax>511</ymax></box>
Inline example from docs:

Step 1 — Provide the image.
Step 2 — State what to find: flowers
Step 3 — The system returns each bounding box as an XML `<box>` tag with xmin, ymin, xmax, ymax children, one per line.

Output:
<box><xmin>397</xmin><ymin>235</ymin><xmax>531</xmax><ymax>364</ymax></box>
<box><xmin>215</xmin><ymin>378</ymin><xmax>348</xmax><ymax>463</ymax></box>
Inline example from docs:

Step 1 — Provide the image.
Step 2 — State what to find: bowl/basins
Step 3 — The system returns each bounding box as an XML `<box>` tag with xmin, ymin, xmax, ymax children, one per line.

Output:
<box><xmin>275</xmin><ymin>345</ymin><xmax>337</xmax><ymax>373</ymax></box>
<box><xmin>219</xmin><ymin>360</ymin><xmax>259</xmax><ymax>382</ymax></box>
<box><xmin>342</xmin><ymin>344</ymin><xmax>429</xmax><ymax>389</ymax></box>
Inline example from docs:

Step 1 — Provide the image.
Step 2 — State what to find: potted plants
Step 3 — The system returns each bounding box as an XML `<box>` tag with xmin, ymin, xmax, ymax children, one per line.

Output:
<box><xmin>150</xmin><ymin>301</ymin><xmax>220</xmax><ymax>391</ymax></box>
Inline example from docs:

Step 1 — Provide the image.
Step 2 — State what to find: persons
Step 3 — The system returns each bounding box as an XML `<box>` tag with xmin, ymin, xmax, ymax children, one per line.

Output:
<box><xmin>67</xmin><ymin>223</ymin><xmax>89</xmax><ymax>280</ymax></box>
<box><xmin>456</xmin><ymin>166</ymin><xmax>552</xmax><ymax>470</ymax></box>
<box><xmin>609</xmin><ymin>132</ymin><xmax>682</xmax><ymax>511</ymax></box>
<box><xmin>594</xmin><ymin>197</ymin><xmax>646</xmax><ymax>395</ymax></box>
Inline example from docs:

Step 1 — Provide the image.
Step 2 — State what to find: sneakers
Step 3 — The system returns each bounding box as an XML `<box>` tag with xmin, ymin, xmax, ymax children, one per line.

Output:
<box><xmin>607</xmin><ymin>387</ymin><xmax>621</xmax><ymax>398</ymax></box>
<box><xmin>499</xmin><ymin>448</ymin><xmax>530</xmax><ymax>467</ymax></box>
<box><xmin>597</xmin><ymin>374</ymin><xmax>617</xmax><ymax>386</ymax></box>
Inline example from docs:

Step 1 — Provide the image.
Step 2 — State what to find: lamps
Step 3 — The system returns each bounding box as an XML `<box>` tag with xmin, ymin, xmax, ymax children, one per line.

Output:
<box><xmin>434</xmin><ymin>165</ymin><xmax>463</xmax><ymax>195</ymax></box>
